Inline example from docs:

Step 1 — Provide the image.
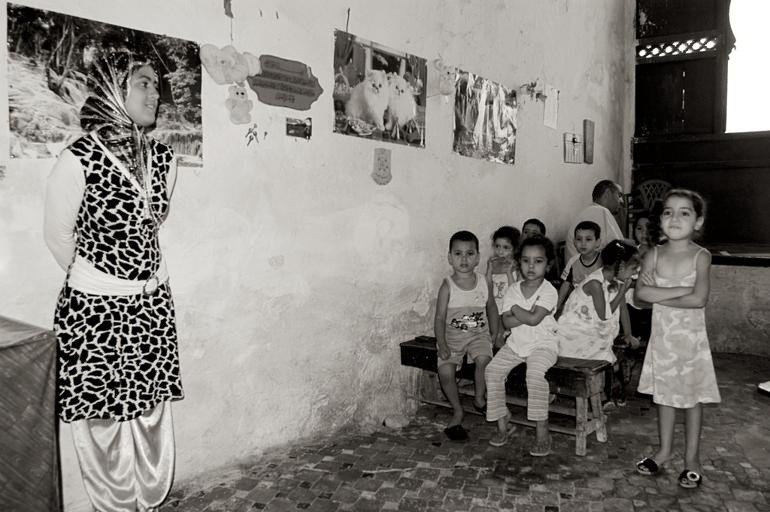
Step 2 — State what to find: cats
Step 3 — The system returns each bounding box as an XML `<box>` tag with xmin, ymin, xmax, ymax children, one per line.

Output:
<box><xmin>388</xmin><ymin>74</ymin><xmax>415</xmax><ymax>139</ymax></box>
<box><xmin>345</xmin><ymin>68</ymin><xmax>387</xmax><ymax>134</ymax></box>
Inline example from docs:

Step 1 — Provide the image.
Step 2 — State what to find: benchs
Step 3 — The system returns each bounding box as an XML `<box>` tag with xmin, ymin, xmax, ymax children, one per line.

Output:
<box><xmin>399</xmin><ymin>334</ymin><xmax>612</xmax><ymax>458</ymax></box>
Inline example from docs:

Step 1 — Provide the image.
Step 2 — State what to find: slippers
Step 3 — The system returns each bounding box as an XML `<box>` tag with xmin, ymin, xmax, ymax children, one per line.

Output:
<box><xmin>489</xmin><ymin>425</ymin><xmax>516</xmax><ymax>446</ymax></box>
<box><xmin>444</xmin><ymin>425</ymin><xmax>468</xmax><ymax>442</ymax></box>
<box><xmin>637</xmin><ymin>454</ymin><xmax>673</xmax><ymax>475</ymax></box>
<box><xmin>530</xmin><ymin>434</ymin><xmax>552</xmax><ymax>456</ymax></box>
<box><xmin>472</xmin><ymin>402</ymin><xmax>486</xmax><ymax>412</ymax></box>
<box><xmin>678</xmin><ymin>470</ymin><xmax>702</xmax><ymax>489</ymax></box>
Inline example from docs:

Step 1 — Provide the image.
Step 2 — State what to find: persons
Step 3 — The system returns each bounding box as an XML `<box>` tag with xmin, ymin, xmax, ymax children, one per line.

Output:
<box><xmin>432</xmin><ymin>178</ymin><xmax>659</xmax><ymax>458</ymax></box>
<box><xmin>39</xmin><ymin>47</ymin><xmax>186</xmax><ymax>512</ymax></box>
<box><xmin>632</xmin><ymin>187</ymin><xmax>723</xmax><ymax>491</ymax></box>
